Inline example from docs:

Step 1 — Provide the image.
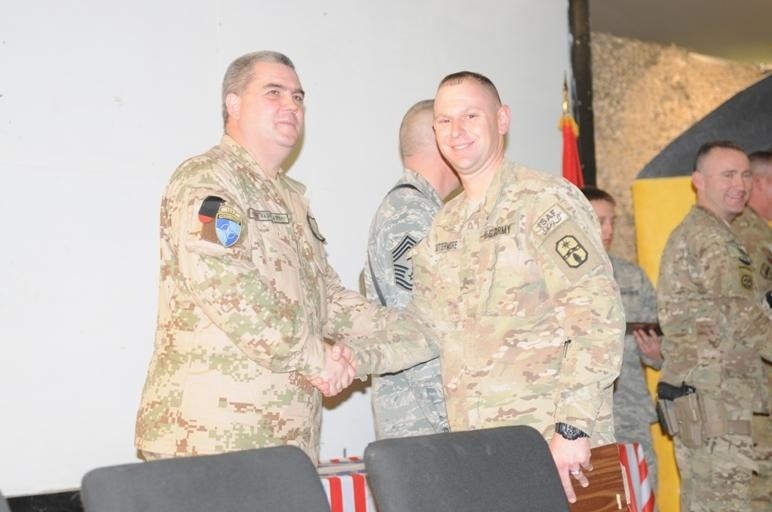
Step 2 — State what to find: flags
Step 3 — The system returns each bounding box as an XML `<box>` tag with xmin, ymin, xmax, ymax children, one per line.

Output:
<box><xmin>556</xmin><ymin>112</ymin><xmax>585</xmax><ymax>191</ymax></box>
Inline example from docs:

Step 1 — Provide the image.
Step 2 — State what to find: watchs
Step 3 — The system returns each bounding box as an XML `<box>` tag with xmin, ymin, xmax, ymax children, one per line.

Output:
<box><xmin>553</xmin><ymin>422</ymin><xmax>590</xmax><ymax>440</ymax></box>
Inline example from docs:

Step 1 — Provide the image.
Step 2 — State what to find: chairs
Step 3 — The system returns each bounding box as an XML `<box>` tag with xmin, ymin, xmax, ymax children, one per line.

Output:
<box><xmin>364</xmin><ymin>425</ymin><xmax>572</xmax><ymax>512</ymax></box>
<box><xmin>78</xmin><ymin>445</ymin><xmax>330</xmax><ymax>511</ymax></box>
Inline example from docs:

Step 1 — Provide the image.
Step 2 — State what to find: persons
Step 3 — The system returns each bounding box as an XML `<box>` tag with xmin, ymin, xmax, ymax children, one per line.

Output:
<box><xmin>363</xmin><ymin>98</ymin><xmax>462</xmax><ymax>441</ymax></box>
<box><xmin>131</xmin><ymin>49</ymin><xmax>386</xmax><ymax>463</ymax></box>
<box><xmin>304</xmin><ymin>68</ymin><xmax>628</xmax><ymax>505</ymax></box>
<box><xmin>729</xmin><ymin>148</ymin><xmax>771</xmax><ymax>305</ymax></box>
<box><xmin>655</xmin><ymin>140</ymin><xmax>772</xmax><ymax>511</ymax></box>
<box><xmin>577</xmin><ymin>185</ymin><xmax>665</xmax><ymax>497</ymax></box>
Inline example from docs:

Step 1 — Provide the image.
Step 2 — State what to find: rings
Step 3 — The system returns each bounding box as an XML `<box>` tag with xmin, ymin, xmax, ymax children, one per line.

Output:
<box><xmin>571</xmin><ymin>468</ymin><xmax>581</xmax><ymax>477</ymax></box>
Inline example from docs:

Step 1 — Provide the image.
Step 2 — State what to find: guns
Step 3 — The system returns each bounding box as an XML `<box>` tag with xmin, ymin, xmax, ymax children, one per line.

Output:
<box><xmin>656</xmin><ymin>381</ymin><xmax>695</xmax><ymax>401</ymax></box>
